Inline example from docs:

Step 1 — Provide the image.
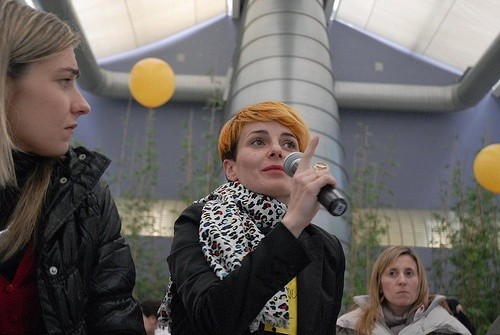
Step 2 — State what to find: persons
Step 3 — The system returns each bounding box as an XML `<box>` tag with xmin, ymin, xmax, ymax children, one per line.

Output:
<box><xmin>0</xmin><ymin>0</ymin><xmax>147</xmax><ymax>335</ymax></box>
<box><xmin>428</xmin><ymin>293</ymin><xmax>500</xmax><ymax>335</ymax></box>
<box><xmin>337</xmin><ymin>245</ymin><xmax>473</xmax><ymax>335</ymax></box>
<box><xmin>153</xmin><ymin>101</ymin><xmax>345</xmax><ymax>335</ymax></box>
<box><xmin>139</xmin><ymin>300</ymin><xmax>163</xmax><ymax>335</ymax></box>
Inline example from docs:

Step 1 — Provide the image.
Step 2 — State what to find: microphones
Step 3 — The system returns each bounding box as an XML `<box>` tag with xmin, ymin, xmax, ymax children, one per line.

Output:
<box><xmin>283</xmin><ymin>152</ymin><xmax>347</xmax><ymax>216</ymax></box>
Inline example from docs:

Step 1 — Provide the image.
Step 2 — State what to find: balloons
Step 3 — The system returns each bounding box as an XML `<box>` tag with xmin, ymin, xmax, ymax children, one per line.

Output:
<box><xmin>474</xmin><ymin>143</ymin><xmax>500</xmax><ymax>194</ymax></box>
<box><xmin>128</xmin><ymin>57</ymin><xmax>177</xmax><ymax>108</ymax></box>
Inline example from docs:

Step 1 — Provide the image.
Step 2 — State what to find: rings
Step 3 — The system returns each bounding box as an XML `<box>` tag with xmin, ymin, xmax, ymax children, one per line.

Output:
<box><xmin>312</xmin><ymin>163</ymin><xmax>329</xmax><ymax>178</ymax></box>
